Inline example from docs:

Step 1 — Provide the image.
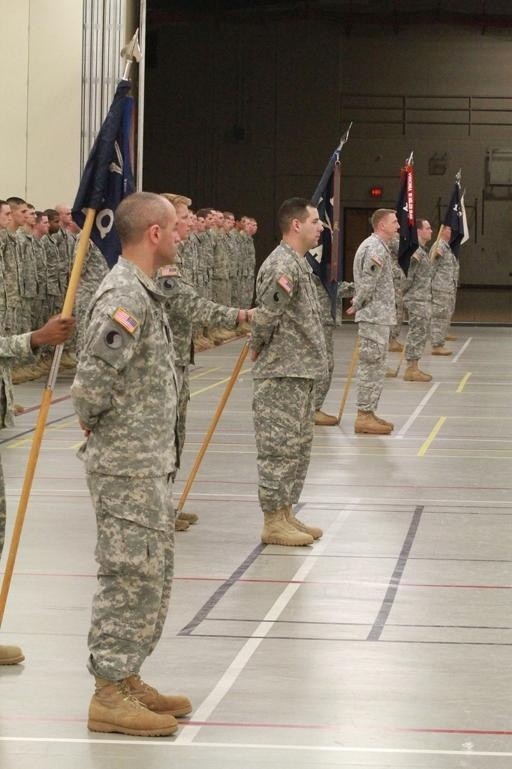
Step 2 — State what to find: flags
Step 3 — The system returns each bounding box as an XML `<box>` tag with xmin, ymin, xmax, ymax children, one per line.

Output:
<box><xmin>392</xmin><ymin>163</ymin><xmax>420</xmax><ymax>275</ymax></box>
<box><xmin>442</xmin><ymin>180</ymin><xmax>471</xmax><ymax>251</ymax></box>
<box><xmin>302</xmin><ymin>151</ymin><xmax>339</xmax><ymax>321</ymax></box>
<box><xmin>69</xmin><ymin>78</ymin><xmax>139</xmax><ymax>271</ymax></box>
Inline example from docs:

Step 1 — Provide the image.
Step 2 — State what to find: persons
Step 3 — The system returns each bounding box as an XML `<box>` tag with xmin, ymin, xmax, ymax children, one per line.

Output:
<box><xmin>313</xmin><ymin>279</ymin><xmax>357</xmax><ymax>426</ymax></box>
<box><xmin>70</xmin><ymin>191</ymin><xmax>194</xmax><ymax>738</ymax></box>
<box><xmin>345</xmin><ymin>208</ymin><xmax>402</xmax><ymax>435</ymax></box>
<box><xmin>444</xmin><ymin>335</ymin><xmax>458</xmax><ymax>343</ymax></box>
<box><xmin>429</xmin><ymin>225</ymin><xmax>460</xmax><ymax>357</ymax></box>
<box><xmin>1</xmin><ymin>196</ymin><xmax>110</xmax><ymax>383</ymax></box>
<box><xmin>395</xmin><ymin>217</ymin><xmax>433</xmax><ymax>382</ymax></box>
<box><xmin>248</xmin><ymin>196</ymin><xmax>332</xmax><ymax>547</ymax></box>
<box><xmin>388</xmin><ymin>232</ymin><xmax>407</xmax><ymax>353</ymax></box>
<box><xmin>177</xmin><ymin>206</ymin><xmax>258</xmax><ymax>353</ymax></box>
<box><xmin>152</xmin><ymin>192</ymin><xmax>259</xmax><ymax>533</ymax></box>
<box><xmin>1</xmin><ymin>313</ymin><xmax>78</xmax><ymax>667</ymax></box>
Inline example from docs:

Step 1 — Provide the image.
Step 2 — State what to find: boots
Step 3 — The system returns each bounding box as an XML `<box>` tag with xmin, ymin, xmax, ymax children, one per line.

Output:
<box><xmin>314</xmin><ymin>409</ymin><xmax>338</xmax><ymax>427</ymax></box>
<box><xmin>174</xmin><ymin>510</ymin><xmax>198</xmax><ymax>530</ymax></box>
<box><xmin>123</xmin><ymin>675</ymin><xmax>193</xmax><ymax>717</ymax></box>
<box><xmin>8</xmin><ymin>353</ymin><xmax>78</xmax><ymax>384</ymax></box>
<box><xmin>192</xmin><ymin>323</ymin><xmax>252</xmax><ymax>350</ymax></box>
<box><xmin>1</xmin><ymin>646</ymin><xmax>24</xmax><ymax>664</ymax></box>
<box><xmin>390</xmin><ymin>335</ymin><xmax>458</xmax><ymax>382</ymax></box>
<box><xmin>262</xmin><ymin>505</ymin><xmax>322</xmax><ymax>545</ymax></box>
<box><xmin>88</xmin><ymin>676</ymin><xmax>179</xmax><ymax>737</ymax></box>
<box><xmin>353</xmin><ymin>409</ymin><xmax>393</xmax><ymax>435</ymax></box>
